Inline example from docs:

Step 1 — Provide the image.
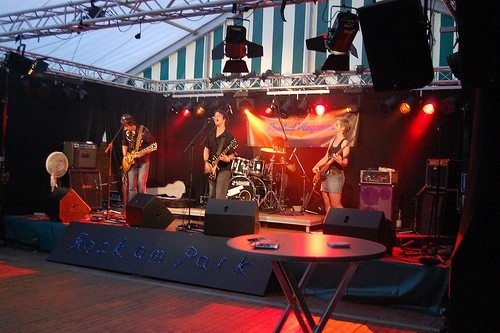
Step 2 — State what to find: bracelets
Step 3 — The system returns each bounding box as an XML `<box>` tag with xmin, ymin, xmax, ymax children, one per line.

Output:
<box><xmin>204</xmin><ymin>160</ymin><xmax>209</xmax><ymax>162</ymax></box>
<box><xmin>123</xmin><ymin>155</ymin><xmax>127</xmax><ymax>157</ymax></box>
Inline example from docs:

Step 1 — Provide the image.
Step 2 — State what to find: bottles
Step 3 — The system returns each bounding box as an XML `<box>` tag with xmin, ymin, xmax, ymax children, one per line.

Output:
<box><xmin>396</xmin><ymin>210</ymin><xmax>402</xmax><ymax>230</ymax></box>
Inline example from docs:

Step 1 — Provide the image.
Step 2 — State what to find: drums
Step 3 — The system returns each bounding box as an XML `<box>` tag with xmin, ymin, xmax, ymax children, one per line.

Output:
<box><xmin>230</xmin><ymin>156</ymin><xmax>252</xmax><ymax>176</ymax></box>
<box><xmin>250</xmin><ymin>158</ymin><xmax>265</xmax><ymax>177</ymax></box>
<box><xmin>226</xmin><ymin>176</ymin><xmax>267</xmax><ymax>205</ymax></box>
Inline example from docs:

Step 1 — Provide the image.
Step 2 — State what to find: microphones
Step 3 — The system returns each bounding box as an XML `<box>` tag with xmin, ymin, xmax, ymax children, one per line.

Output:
<box><xmin>229</xmin><ymin>105</ymin><xmax>233</xmax><ymax>114</ymax></box>
<box><xmin>208</xmin><ymin>117</ymin><xmax>216</xmax><ymax>120</ymax></box>
<box><xmin>289</xmin><ymin>148</ymin><xmax>297</xmax><ymax>160</ymax></box>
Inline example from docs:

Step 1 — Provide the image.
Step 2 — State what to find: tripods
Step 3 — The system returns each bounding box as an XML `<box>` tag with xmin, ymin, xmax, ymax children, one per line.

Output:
<box><xmin>258</xmin><ymin>154</ymin><xmax>295</xmax><ymax>212</ymax></box>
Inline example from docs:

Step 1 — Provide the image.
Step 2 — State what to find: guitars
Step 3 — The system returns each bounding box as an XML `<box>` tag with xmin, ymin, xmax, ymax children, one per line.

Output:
<box><xmin>122</xmin><ymin>142</ymin><xmax>157</xmax><ymax>172</ymax></box>
<box><xmin>312</xmin><ymin>136</ymin><xmax>357</xmax><ymax>187</ymax></box>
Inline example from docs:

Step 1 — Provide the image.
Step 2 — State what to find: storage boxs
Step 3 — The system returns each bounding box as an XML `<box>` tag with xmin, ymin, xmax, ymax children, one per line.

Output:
<box><xmin>360</xmin><ymin>170</ymin><xmax>398</xmax><ymax>184</ymax></box>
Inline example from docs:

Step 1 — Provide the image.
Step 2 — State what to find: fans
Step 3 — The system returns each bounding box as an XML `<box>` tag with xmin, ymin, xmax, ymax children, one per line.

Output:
<box><xmin>45</xmin><ymin>152</ymin><xmax>69</xmax><ymax>192</ymax></box>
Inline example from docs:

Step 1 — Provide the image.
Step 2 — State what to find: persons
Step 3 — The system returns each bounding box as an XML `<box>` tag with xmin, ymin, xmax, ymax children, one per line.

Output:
<box><xmin>120</xmin><ymin>114</ymin><xmax>152</xmax><ymax>202</ymax></box>
<box><xmin>202</xmin><ymin>109</ymin><xmax>237</xmax><ymax>202</ymax></box>
<box><xmin>312</xmin><ymin>117</ymin><xmax>351</xmax><ymax>209</ymax></box>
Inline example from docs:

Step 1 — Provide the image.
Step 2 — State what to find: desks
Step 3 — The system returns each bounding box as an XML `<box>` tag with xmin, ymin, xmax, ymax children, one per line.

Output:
<box><xmin>227</xmin><ymin>231</ymin><xmax>387</xmax><ymax>333</ymax></box>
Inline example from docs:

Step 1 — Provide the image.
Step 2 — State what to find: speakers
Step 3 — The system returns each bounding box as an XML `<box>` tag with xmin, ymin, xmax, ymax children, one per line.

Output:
<box><xmin>126</xmin><ymin>193</ymin><xmax>176</xmax><ymax>230</ymax></box>
<box><xmin>357</xmin><ymin>0</ymin><xmax>434</xmax><ymax>94</ymax></box>
<box><xmin>417</xmin><ymin>159</ymin><xmax>466</xmax><ymax>247</ymax></box>
<box><xmin>4</xmin><ymin>51</ymin><xmax>33</xmax><ymax>74</ymax></box>
<box><xmin>322</xmin><ymin>185</ymin><xmax>400</xmax><ymax>245</ymax></box>
<box><xmin>45</xmin><ymin>140</ymin><xmax>102</xmax><ymax>223</ymax></box>
<box><xmin>204</xmin><ymin>199</ymin><xmax>261</xmax><ymax>237</ymax></box>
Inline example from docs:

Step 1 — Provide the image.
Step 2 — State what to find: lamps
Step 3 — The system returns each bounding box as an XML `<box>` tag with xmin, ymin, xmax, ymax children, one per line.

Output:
<box><xmin>306</xmin><ymin>5</ymin><xmax>359</xmax><ymax>71</ymax></box>
<box><xmin>38</xmin><ymin>81</ymin><xmax>89</xmax><ymax>103</ymax></box>
<box><xmin>180</xmin><ymin>99</ymin><xmax>224</xmax><ymax>118</ymax></box>
<box><xmin>267</xmin><ymin>95</ymin><xmax>311</xmax><ymax>118</ymax></box>
<box><xmin>211</xmin><ymin>18</ymin><xmax>263</xmax><ymax>74</ymax></box>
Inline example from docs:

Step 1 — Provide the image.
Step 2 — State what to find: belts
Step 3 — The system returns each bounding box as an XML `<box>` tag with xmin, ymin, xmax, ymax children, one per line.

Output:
<box><xmin>326</xmin><ymin>170</ymin><xmax>344</xmax><ymax>174</ymax></box>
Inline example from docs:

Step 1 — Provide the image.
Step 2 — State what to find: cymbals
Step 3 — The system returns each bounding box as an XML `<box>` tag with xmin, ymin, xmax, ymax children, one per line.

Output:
<box><xmin>274</xmin><ymin>162</ymin><xmax>290</xmax><ymax>165</ymax></box>
<box><xmin>259</xmin><ymin>148</ymin><xmax>286</xmax><ymax>154</ymax></box>
